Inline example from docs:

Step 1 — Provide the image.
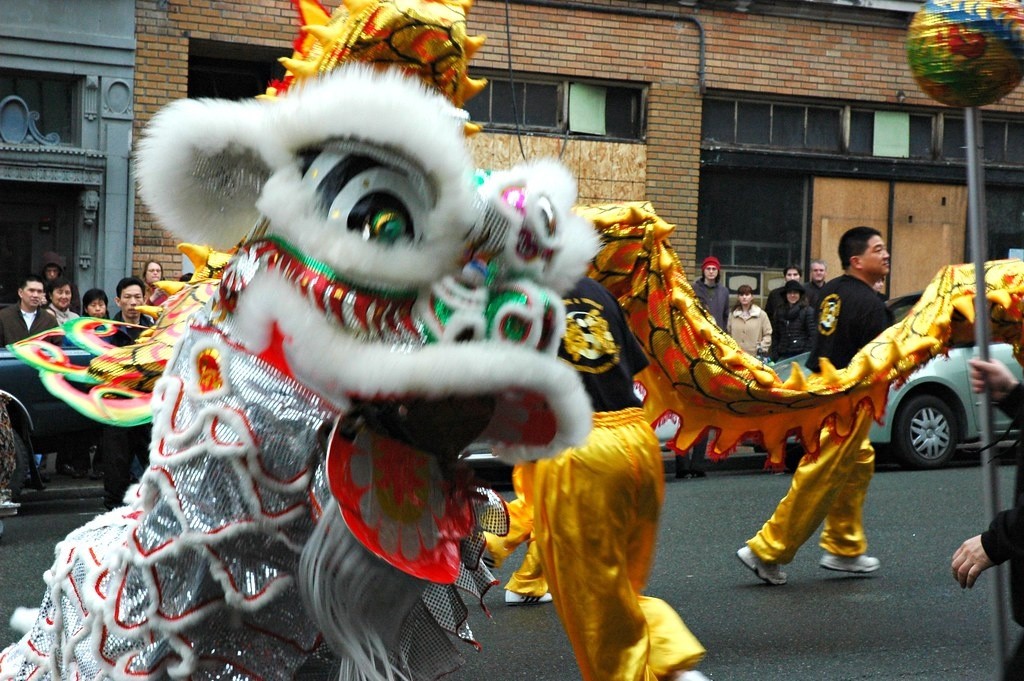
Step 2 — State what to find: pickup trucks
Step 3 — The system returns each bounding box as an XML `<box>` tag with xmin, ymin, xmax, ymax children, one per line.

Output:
<box><xmin>0</xmin><ymin>345</ymin><xmax>103</xmax><ymax>497</ymax></box>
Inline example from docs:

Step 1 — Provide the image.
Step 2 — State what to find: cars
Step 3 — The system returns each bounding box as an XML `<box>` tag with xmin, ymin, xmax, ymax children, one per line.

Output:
<box><xmin>770</xmin><ymin>287</ymin><xmax>1024</xmax><ymax>467</ymax></box>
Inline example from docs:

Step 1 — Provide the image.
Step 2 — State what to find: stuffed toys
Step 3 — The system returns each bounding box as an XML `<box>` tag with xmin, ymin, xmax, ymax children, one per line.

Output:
<box><xmin>0</xmin><ymin>0</ymin><xmax>605</xmax><ymax>681</ymax></box>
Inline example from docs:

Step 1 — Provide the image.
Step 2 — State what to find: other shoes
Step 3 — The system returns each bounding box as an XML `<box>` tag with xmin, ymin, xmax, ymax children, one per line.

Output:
<box><xmin>89</xmin><ymin>470</ymin><xmax>105</xmax><ymax>481</ymax></box>
<box><xmin>56</xmin><ymin>463</ymin><xmax>72</xmax><ymax>475</ymax></box>
<box><xmin>24</xmin><ymin>478</ymin><xmax>32</xmax><ymax>488</ymax></box>
<box><xmin>103</xmin><ymin>502</ymin><xmax>126</xmax><ymax>511</ymax></box>
<box><xmin>38</xmin><ymin>466</ymin><xmax>50</xmax><ymax>483</ymax></box>
<box><xmin>72</xmin><ymin>468</ymin><xmax>87</xmax><ymax>479</ymax></box>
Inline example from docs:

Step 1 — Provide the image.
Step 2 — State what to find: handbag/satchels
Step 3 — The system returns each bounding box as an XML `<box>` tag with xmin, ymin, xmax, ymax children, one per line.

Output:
<box><xmin>757</xmin><ymin>349</ymin><xmax>769</xmax><ymax>364</ymax></box>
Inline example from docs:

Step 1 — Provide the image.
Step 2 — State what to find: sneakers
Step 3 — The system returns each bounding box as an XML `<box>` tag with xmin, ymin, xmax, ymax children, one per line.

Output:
<box><xmin>483</xmin><ymin>550</ymin><xmax>495</xmax><ymax>572</ymax></box>
<box><xmin>819</xmin><ymin>548</ymin><xmax>880</xmax><ymax>572</ymax></box>
<box><xmin>737</xmin><ymin>545</ymin><xmax>787</xmax><ymax>584</ymax></box>
<box><xmin>505</xmin><ymin>587</ymin><xmax>553</xmax><ymax>605</ymax></box>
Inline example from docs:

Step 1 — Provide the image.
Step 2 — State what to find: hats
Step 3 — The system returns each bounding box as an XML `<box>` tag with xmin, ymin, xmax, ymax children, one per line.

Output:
<box><xmin>701</xmin><ymin>256</ymin><xmax>721</xmax><ymax>273</ymax></box>
<box><xmin>780</xmin><ymin>280</ymin><xmax>805</xmax><ymax>300</ymax></box>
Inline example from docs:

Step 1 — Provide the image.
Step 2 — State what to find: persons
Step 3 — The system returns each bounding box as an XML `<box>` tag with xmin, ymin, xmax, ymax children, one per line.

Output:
<box><xmin>675</xmin><ymin>256</ymin><xmax>827</xmax><ymax>480</ymax></box>
<box><xmin>951</xmin><ymin>357</ymin><xmax>1024</xmax><ymax>681</ymax></box>
<box><xmin>531</xmin><ymin>275</ymin><xmax>711</xmax><ymax>681</ymax></box>
<box><xmin>0</xmin><ymin>252</ymin><xmax>196</xmax><ymax>515</ymax></box>
<box><xmin>736</xmin><ymin>227</ymin><xmax>891</xmax><ymax>585</ymax></box>
<box><xmin>480</xmin><ymin>460</ymin><xmax>553</xmax><ymax>607</ymax></box>
